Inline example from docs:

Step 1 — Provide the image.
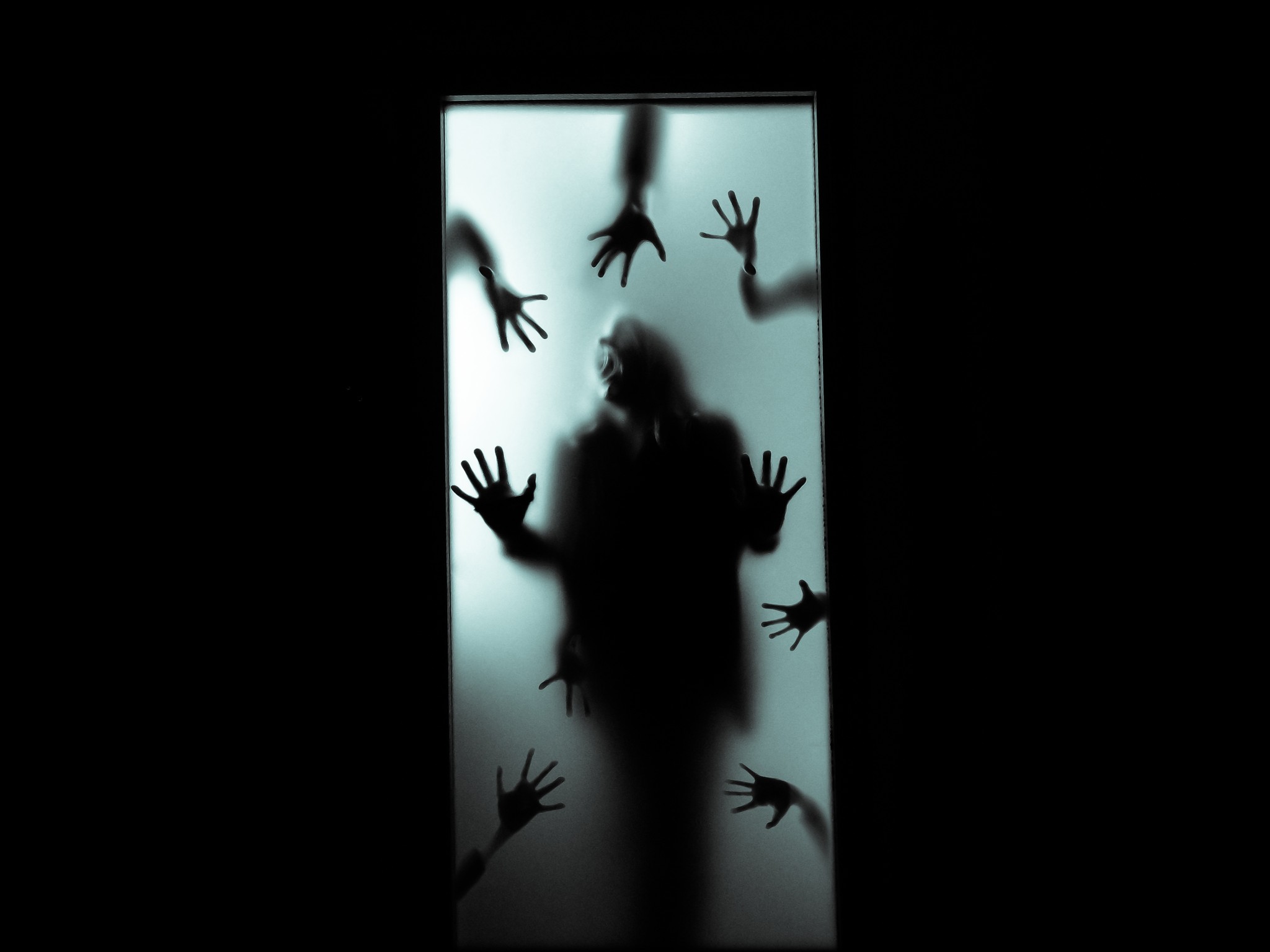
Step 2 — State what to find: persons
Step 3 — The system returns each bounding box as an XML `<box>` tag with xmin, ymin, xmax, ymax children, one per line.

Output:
<box><xmin>443</xmin><ymin>103</ymin><xmax>832</xmax><ymax>952</ymax></box>
